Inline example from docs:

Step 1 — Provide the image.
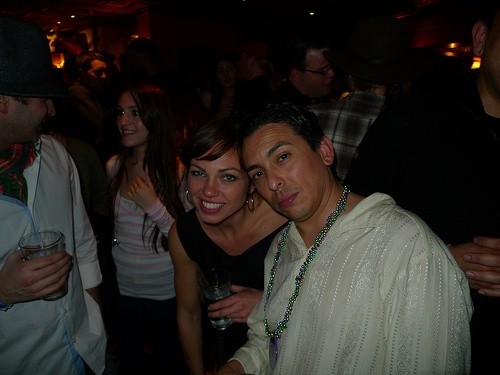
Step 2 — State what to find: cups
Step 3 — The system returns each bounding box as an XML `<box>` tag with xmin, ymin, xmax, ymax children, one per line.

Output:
<box><xmin>199</xmin><ymin>267</ymin><xmax>234</xmax><ymax>330</ymax></box>
<box><xmin>18</xmin><ymin>229</ymin><xmax>68</xmax><ymax>301</ymax></box>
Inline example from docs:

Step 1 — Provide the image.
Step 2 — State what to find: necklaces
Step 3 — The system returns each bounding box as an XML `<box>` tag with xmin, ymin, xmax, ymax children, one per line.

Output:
<box><xmin>264</xmin><ymin>185</ymin><xmax>350</xmax><ymax>366</ymax></box>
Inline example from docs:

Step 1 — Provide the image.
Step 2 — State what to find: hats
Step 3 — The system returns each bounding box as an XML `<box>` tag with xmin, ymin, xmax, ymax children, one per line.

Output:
<box><xmin>0</xmin><ymin>12</ymin><xmax>64</xmax><ymax>97</ymax></box>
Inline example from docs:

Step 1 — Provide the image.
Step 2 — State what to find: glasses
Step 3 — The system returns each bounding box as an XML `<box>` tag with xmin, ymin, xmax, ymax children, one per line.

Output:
<box><xmin>296</xmin><ymin>58</ymin><xmax>336</xmax><ymax>77</ymax></box>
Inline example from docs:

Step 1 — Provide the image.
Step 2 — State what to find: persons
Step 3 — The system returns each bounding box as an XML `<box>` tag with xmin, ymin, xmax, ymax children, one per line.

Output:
<box><xmin>0</xmin><ymin>18</ymin><xmax>106</xmax><ymax>375</ymax></box>
<box><xmin>0</xmin><ymin>0</ymin><xmax>460</xmax><ymax>214</ymax></box>
<box><xmin>170</xmin><ymin>119</ymin><xmax>288</xmax><ymax>375</ymax></box>
<box><xmin>108</xmin><ymin>86</ymin><xmax>195</xmax><ymax>375</ymax></box>
<box><xmin>223</xmin><ymin>103</ymin><xmax>473</xmax><ymax>375</ymax></box>
<box><xmin>346</xmin><ymin>0</ymin><xmax>500</xmax><ymax>375</ymax></box>
